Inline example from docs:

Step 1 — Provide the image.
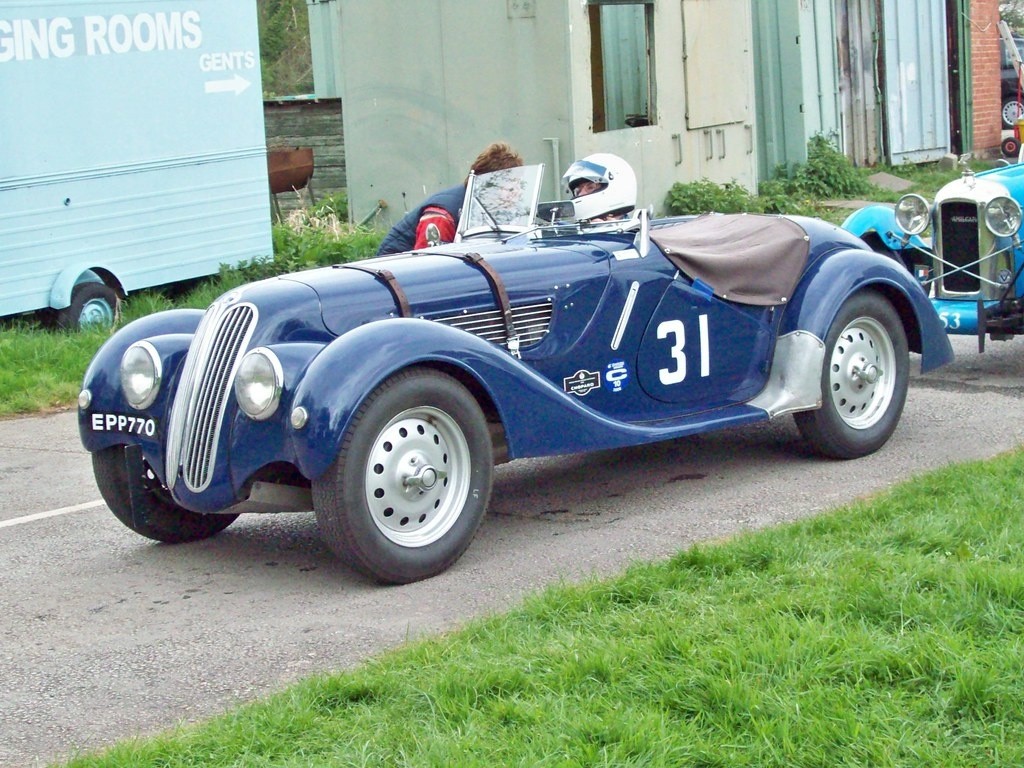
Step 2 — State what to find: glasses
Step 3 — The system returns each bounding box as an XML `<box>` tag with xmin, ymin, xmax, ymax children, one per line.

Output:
<box><xmin>574</xmin><ymin>182</ymin><xmax>602</xmax><ymax>198</ymax></box>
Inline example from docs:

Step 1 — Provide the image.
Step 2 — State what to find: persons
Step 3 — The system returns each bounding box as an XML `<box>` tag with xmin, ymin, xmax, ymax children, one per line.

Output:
<box><xmin>556</xmin><ymin>153</ymin><xmax>636</xmax><ymax>226</ymax></box>
<box><xmin>378</xmin><ymin>142</ymin><xmax>524</xmax><ymax>257</ymax></box>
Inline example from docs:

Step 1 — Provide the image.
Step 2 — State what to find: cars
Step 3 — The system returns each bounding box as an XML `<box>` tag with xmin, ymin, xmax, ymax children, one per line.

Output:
<box><xmin>949</xmin><ymin>17</ymin><xmax>1023</xmax><ymax>130</ymax></box>
<box><xmin>840</xmin><ymin>143</ymin><xmax>1024</xmax><ymax>353</ymax></box>
<box><xmin>72</xmin><ymin>163</ymin><xmax>957</xmax><ymax>586</ymax></box>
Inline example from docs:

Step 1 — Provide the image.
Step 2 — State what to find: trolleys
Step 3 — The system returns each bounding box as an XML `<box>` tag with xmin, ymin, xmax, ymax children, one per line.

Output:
<box><xmin>1000</xmin><ymin>57</ymin><xmax>1024</xmax><ymax>159</ymax></box>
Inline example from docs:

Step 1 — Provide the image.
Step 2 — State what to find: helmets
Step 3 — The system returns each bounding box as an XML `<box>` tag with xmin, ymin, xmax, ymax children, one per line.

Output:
<box><xmin>563</xmin><ymin>152</ymin><xmax>637</xmax><ymax>222</ymax></box>
